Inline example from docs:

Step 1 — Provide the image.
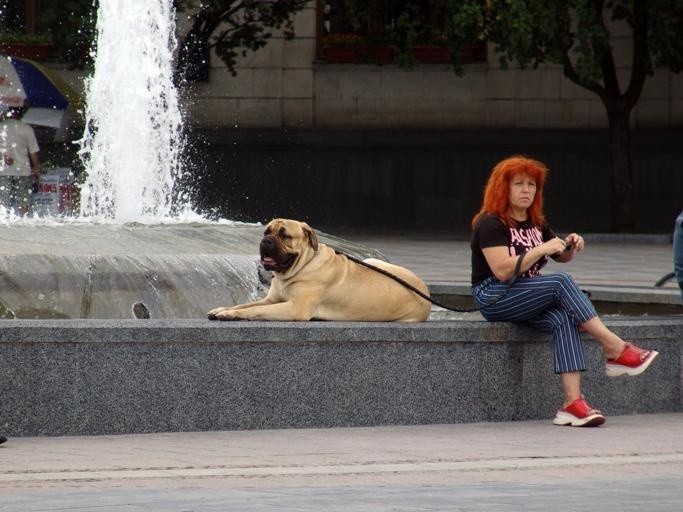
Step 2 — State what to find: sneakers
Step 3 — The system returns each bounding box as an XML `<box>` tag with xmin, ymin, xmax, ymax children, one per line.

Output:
<box><xmin>605</xmin><ymin>343</ymin><xmax>659</xmax><ymax>376</ymax></box>
<box><xmin>553</xmin><ymin>396</ymin><xmax>606</xmax><ymax>426</ymax></box>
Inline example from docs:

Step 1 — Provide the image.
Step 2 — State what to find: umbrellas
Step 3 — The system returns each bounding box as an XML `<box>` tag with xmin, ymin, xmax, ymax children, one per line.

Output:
<box><xmin>0</xmin><ymin>54</ymin><xmax>87</xmax><ymax>112</ymax></box>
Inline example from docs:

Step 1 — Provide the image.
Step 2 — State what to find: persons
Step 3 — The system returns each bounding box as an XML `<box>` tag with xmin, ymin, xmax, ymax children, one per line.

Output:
<box><xmin>470</xmin><ymin>154</ymin><xmax>661</xmax><ymax>428</ymax></box>
<box><xmin>0</xmin><ymin>106</ymin><xmax>42</xmax><ymax>216</ymax></box>
<box><xmin>672</xmin><ymin>211</ymin><xmax>683</xmax><ymax>294</ymax></box>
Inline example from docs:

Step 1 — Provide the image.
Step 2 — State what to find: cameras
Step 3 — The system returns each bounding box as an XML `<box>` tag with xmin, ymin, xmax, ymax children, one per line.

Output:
<box><xmin>564</xmin><ymin>241</ymin><xmax>574</xmax><ymax>253</ymax></box>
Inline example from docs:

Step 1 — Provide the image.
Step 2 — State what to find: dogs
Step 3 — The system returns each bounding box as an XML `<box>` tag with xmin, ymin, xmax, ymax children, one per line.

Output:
<box><xmin>205</xmin><ymin>218</ymin><xmax>432</xmax><ymax>321</ymax></box>
